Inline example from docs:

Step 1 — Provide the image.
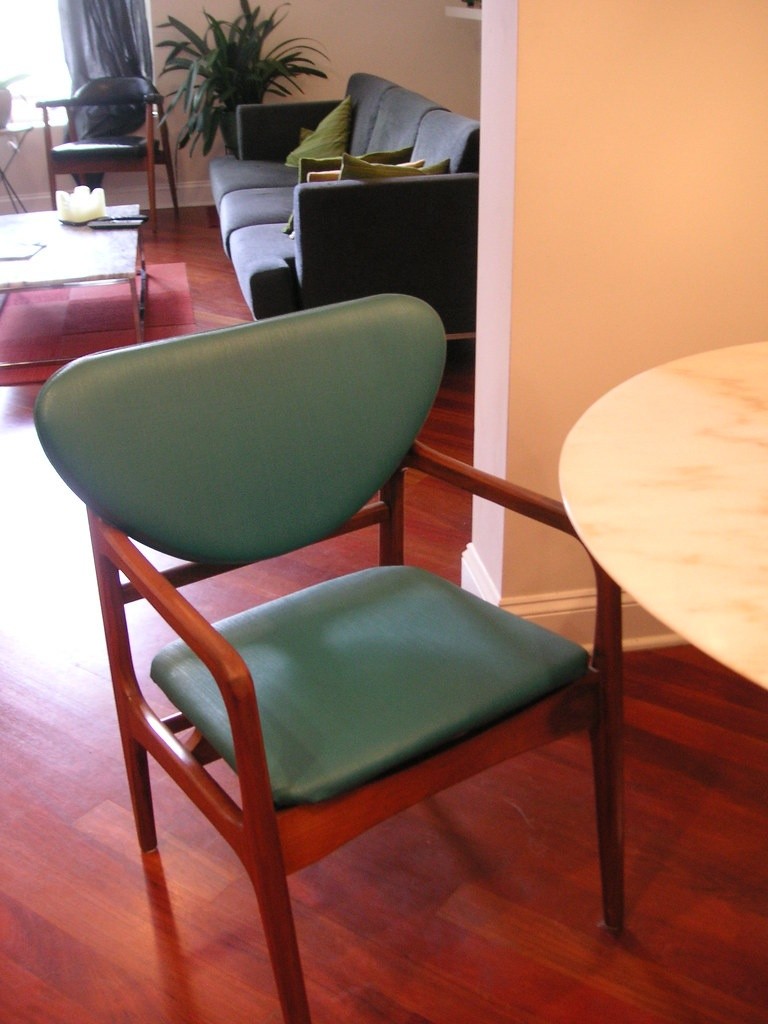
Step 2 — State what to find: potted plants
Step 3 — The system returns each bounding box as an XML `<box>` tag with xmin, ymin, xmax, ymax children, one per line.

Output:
<box><xmin>156</xmin><ymin>0</ymin><xmax>331</xmax><ymax>157</ymax></box>
<box><xmin>0</xmin><ymin>72</ymin><xmax>29</xmax><ymax>130</ymax></box>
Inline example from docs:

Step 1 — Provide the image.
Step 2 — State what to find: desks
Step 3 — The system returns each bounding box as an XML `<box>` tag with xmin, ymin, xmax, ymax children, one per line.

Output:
<box><xmin>557</xmin><ymin>341</ymin><xmax>768</xmax><ymax>690</ymax></box>
<box><xmin>0</xmin><ymin>124</ymin><xmax>33</xmax><ymax>213</ymax></box>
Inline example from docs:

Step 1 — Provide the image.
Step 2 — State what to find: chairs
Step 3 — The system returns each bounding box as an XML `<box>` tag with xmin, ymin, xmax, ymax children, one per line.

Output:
<box><xmin>33</xmin><ymin>294</ymin><xmax>625</xmax><ymax>1024</ymax></box>
<box><xmin>36</xmin><ymin>76</ymin><xmax>179</xmax><ymax>234</ymax></box>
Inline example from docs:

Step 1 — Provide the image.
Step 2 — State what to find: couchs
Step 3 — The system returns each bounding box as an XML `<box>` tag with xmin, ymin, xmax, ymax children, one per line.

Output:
<box><xmin>208</xmin><ymin>71</ymin><xmax>480</xmax><ymax>321</ymax></box>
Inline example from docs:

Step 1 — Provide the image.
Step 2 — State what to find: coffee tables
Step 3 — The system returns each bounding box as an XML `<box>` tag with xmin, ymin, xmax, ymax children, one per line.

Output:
<box><xmin>0</xmin><ymin>204</ymin><xmax>147</xmax><ymax>369</ymax></box>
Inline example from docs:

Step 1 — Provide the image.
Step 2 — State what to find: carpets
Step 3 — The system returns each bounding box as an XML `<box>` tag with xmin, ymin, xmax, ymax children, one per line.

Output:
<box><xmin>0</xmin><ymin>262</ymin><xmax>194</xmax><ymax>386</ymax></box>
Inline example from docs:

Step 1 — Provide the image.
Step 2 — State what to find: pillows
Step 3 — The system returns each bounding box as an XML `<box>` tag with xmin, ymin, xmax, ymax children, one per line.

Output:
<box><xmin>289</xmin><ymin>159</ymin><xmax>425</xmax><ymax>239</ymax></box>
<box><xmin>283</xmin><ymin>145</ymin><xmax>413</xmax><ymax>234</ymax></box>
<box><xmin>284</xmin><ymin>95</ymin><xmax>352</xmax><ymax>167</ymax></box>
<box><xmin>337</xmin><ymin>153</ymin><xmax>451</xmax><ymax>180</ymax></box>
